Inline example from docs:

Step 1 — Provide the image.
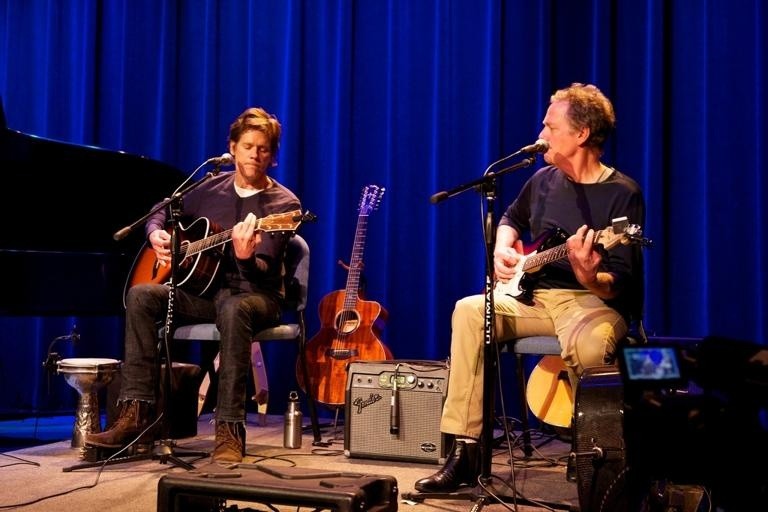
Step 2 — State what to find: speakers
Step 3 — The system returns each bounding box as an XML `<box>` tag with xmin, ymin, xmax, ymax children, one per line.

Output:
<box><xmin>157</xmin><ymin>462</ymin><xmax>398</xmax><ymax>512</ymax></box>
<box><xmin>107</xmin><ymin>362</ymin><xmax>201</xmax><ymax>443</ymax></box>
<box><xmin>344</xmin><ymin>360</ymin><xmax>447</xmax><ymax>464</ymax></box>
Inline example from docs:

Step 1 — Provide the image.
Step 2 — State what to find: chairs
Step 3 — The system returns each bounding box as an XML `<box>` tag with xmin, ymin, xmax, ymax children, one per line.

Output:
<box><xmin>495</xmin><ymin>336</ymin><xmax>576</xmax><ymax>462</ymax></box>
<box><xmin>156</xmin><ymin>232</ymin><xmax>321</xmax><ymax>448</ymax></box>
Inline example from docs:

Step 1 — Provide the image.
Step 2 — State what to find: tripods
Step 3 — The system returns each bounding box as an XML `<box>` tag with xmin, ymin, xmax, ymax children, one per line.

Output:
<box><xmin>400</xmin><ymin>156</ymin><xmax>579</xmax><ymax>512</ymax></box>
<box><xmin>62</xmin><ymin>165</ymin><xmax>211</xmax><ymax>472</ymax></box>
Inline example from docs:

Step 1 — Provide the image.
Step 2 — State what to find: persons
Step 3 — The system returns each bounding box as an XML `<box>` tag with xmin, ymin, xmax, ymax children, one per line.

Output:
<box><xmin>415</xmin><ymin>83</ymin><xmax>645</xmax><ymax>493</ymax></box>
<box><xmin>85</xmin><ymin>108</ymin><xmax>302</xmax><ymax>463</ymax></box>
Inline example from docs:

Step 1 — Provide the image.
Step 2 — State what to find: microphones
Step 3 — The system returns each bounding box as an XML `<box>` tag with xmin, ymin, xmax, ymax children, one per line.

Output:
<box><xmin>521</xmin><ymin>139</ymin><xmax>551</xmax><ymax>154</ymax></box>
<box><xmin>208</xmin><ymin>153</ymin><xmax>233</xmax><ymax>165</ymax></box>
<box><xmin>390</xmin><ymin>379</ymin><xmax>400</xmax><ymax>434</ymax></box>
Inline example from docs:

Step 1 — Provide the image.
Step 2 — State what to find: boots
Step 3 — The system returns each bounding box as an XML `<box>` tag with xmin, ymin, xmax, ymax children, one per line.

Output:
<box><xmin>210</xmin><ymin>421</ymin><xmax>242</xmax><ymax>469</ymax></box>
<box><xmin>85</xmin><ymin>400</ymin><xmax>151</xmax><ymax>453</ymax></box>
<box><xmin>416</xmin><ymin>440</ymin><xmax>479</xmax><ymax>493</ymax></box>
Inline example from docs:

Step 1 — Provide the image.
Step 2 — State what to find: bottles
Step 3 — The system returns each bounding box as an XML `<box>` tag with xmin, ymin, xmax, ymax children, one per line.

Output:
<box><xmin>283</xmin><ymin>389</ymin><xmax>304</xmax><ymax>449</ymax></box>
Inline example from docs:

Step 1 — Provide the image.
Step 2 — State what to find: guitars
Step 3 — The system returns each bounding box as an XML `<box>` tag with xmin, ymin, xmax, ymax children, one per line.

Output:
<box><xmin>122</xmin><ymin>210</ymin><xmax>316</xmax><ymax>307</ymax></box>
<box><xmin>297</xmin><ymin>186</ymin><xmax>396</xmax><ymax>405</ymax></box>
<box><xmin>492</xmin><ymin>215</ymin><xmax>653</xmax><ymax>299</ymax></box>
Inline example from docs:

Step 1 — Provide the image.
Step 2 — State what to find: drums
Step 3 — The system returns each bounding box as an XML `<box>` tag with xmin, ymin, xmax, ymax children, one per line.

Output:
<box><xmin>57</xmin><ymin>358</ymin><xmax>118</xmax><ymax>448</ymax></box>
